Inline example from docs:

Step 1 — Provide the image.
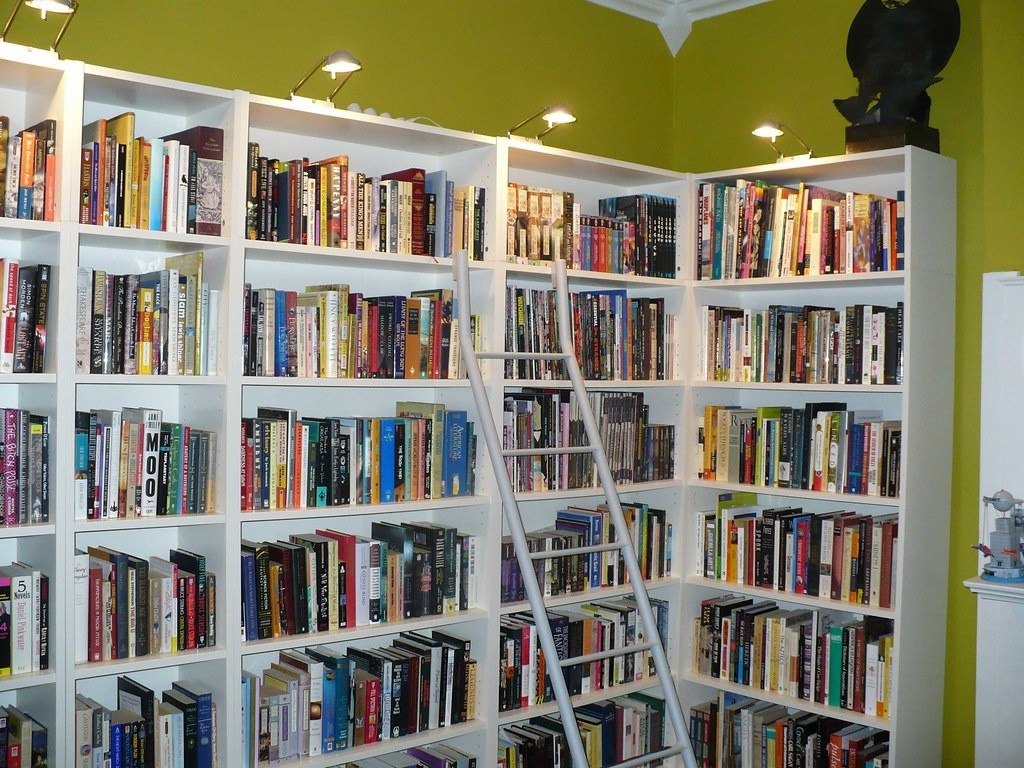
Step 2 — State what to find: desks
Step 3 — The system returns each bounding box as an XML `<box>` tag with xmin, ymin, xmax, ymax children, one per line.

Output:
<box><xmin>963</xmin><ymin>574</ymin><xmax>1024</xmax><ymax>768</ymax></box>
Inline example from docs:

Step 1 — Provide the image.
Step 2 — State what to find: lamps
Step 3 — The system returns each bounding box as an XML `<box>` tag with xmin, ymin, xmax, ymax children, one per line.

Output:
<box><xmin>289</xmin><ymin>48</ymin><xmax>362</xmax><ymax>100</ymax></box>
<box><xmin>506</xmin><ymin>103</ymin><xmax>577</xmax><ymax>140</ymax></box>
<box><xmin>751</xmin><ymin>118</ymin><xmax>816</xmax><ymax>158</ymax></box>
<box><xmin>0</xmin><ymin>0</ymin><xmax>79</xmax><ymax>52</ymax></box>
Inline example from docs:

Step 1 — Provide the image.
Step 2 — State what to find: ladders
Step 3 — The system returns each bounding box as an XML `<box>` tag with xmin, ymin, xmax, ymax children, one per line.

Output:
<box><xmin>453</xmin><ymin>250</ymin><xmax>698</xmax><ymax>768</ymax></box>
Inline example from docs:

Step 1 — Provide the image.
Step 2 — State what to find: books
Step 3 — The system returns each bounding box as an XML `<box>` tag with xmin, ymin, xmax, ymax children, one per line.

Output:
<box><xmin>2</xmin><ymin>110</ymin><xmax>677</xmax><ymax>768</ymax></box>
<box><xmin>687</xmin><ymin>179</ymin><xmax>905</xmax><ymax>768</ymax></box>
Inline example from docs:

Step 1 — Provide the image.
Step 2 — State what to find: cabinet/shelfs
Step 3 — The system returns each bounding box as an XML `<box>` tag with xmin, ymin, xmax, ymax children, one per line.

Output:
<box><xmin>675</xmin><ymin>143</ymin><xmax>958</xmax><ymax>768</ymax></box>
<box><xmin>0</xmin><ymin>48</ymin><xmax>692</xmax><ymax>768</ymax></box>
